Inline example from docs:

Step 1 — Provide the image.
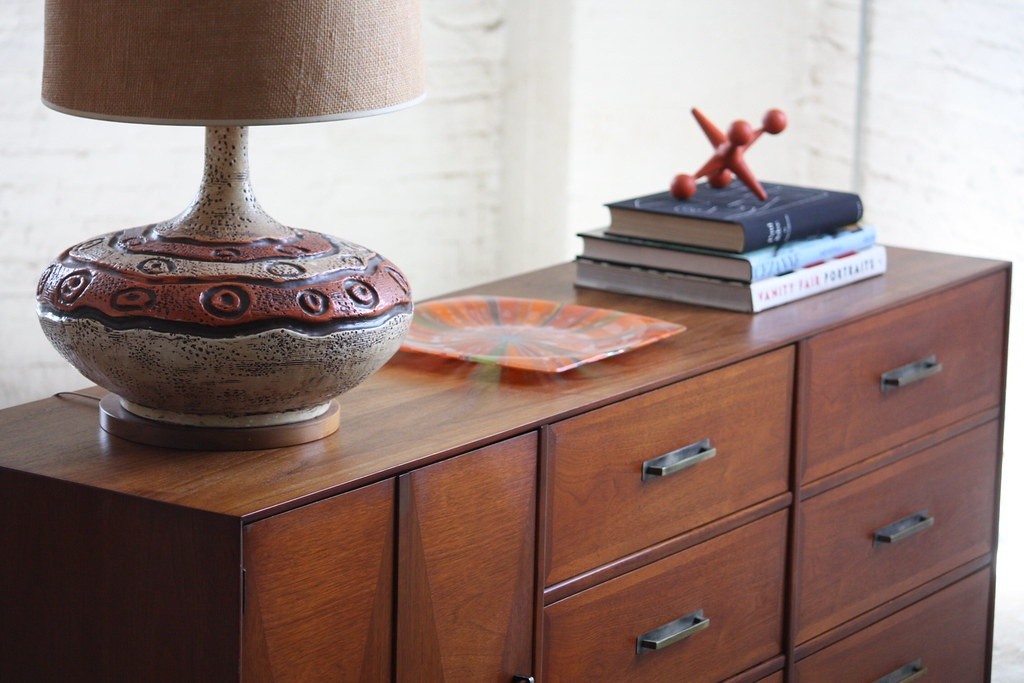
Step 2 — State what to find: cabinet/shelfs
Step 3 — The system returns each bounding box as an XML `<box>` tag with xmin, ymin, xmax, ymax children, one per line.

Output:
<box><xmin>0</xmin><ymin>242</ymin><xmax>1013</xmax><ymax>683</ymax></box>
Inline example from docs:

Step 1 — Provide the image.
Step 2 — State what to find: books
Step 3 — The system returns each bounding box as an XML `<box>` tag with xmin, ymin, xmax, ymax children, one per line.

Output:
<box><xmin>571</xmin><ymin>175</ymin><xmax>887</xmax><ymax>314</ymax></box>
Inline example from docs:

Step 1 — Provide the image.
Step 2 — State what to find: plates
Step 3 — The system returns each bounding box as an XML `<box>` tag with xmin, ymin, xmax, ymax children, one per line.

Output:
<box><xmin>398</xmin><ymin>293</ymin><xmax>686</xmax><ymax>375</ymax></box>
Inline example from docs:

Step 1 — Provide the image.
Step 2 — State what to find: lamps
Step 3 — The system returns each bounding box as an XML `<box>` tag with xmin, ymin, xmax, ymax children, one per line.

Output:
<box><xmin>38</xmin><ymin>0</ymin><xmax>430</xmax><ymax>449</ymax></box>
